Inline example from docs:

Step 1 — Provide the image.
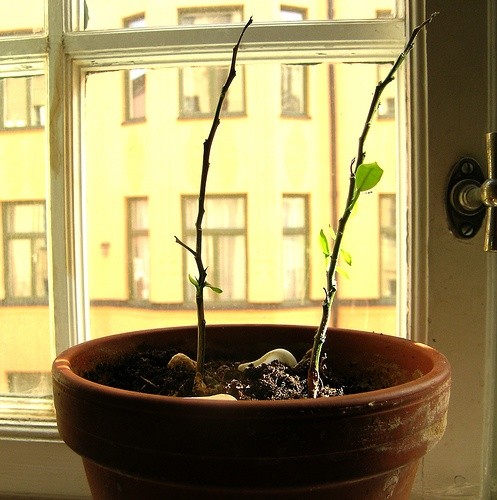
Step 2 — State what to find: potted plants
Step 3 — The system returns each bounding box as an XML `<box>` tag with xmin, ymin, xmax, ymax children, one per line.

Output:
<box><xmin>53</xmin><ymin>8</ymin><xmax>453</xmax><ymax>500</ymax></box>
